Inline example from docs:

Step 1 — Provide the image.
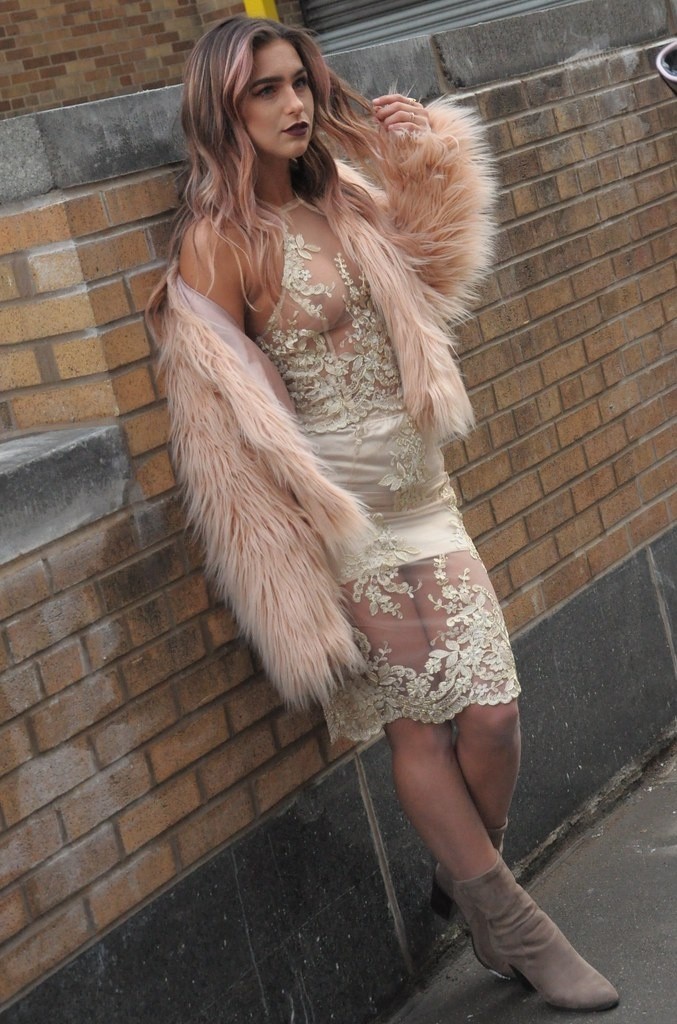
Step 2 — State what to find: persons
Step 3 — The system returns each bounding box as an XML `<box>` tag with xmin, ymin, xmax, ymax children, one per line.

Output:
<box><xmin>143</xmin><ymin>15</ymin><xmax>625</xmax><ymax>1013</ymax></box>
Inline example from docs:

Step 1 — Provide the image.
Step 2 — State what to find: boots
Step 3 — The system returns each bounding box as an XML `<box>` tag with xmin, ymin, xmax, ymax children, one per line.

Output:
<box><xmin>429</xmin><ymin>816</ymin><xmax>621</xmax><ymax>1013</ymax></box>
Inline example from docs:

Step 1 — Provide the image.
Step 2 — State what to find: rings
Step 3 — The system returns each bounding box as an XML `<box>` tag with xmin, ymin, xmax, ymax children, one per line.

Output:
<box><xmin>409</xmin><ymin>97</ymin><xmax>416</xmax><ymax>104</ymax></box>
<box><xmin>409</xmin><ymin>113</ymin><xmax>414</xmax><ymax>122</ymax></box>
